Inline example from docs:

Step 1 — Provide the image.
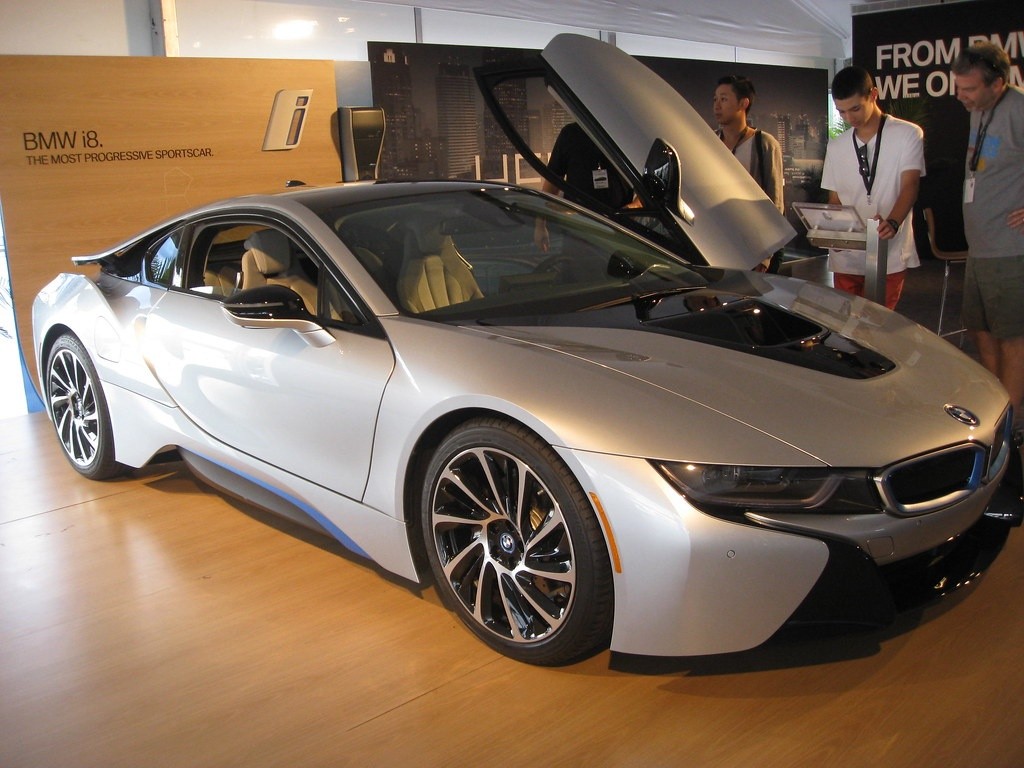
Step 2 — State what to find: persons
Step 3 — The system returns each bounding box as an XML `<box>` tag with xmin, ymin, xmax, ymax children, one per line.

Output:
<box><xmin>534</xmin><ymin>122</ymin><xmax>643</xmax><ymax>286</ymax></box>
<box><xmin>949</xmin><ymin>41</ymin><xmax>1024</xmax><ymax>433</ymax></box>
<box><xmin>820</xmin><ymin>66</ymin><xmax>927</xmax><ymax>311</ymax></box>
<box><xmin>711</xmin><ymin>75</ymin><xmax>786</xmax><ymax>274</ymax></box>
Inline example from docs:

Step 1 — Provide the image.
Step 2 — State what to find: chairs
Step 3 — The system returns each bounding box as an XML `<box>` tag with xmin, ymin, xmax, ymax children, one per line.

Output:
<box><xmin>397</xmin><ymin>212</ymin><xmax>485</xmax><ymax>313</ymax></box>
<box><xmin>922</xmin><ymin>202</ymin><xmax>970</xmax><ymax>351</ymax></box>
<box><xmin>241</xmin><ymin>227</ymin><xmax>343</xmax><ymax>325</ymax></box>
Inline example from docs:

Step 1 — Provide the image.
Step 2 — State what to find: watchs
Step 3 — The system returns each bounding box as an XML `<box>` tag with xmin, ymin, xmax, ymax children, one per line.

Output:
<box><xmin>886</xmin><ymin>219</ymin><xmax>899</xmax><ymax>234</ymax></box>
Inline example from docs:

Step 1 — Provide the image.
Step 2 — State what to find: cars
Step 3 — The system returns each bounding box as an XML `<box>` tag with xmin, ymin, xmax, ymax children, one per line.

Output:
<box><xmin>31</xmin><ymin>32</ymin><xmax>1012</xmax><ymax>675</ymax></box>
